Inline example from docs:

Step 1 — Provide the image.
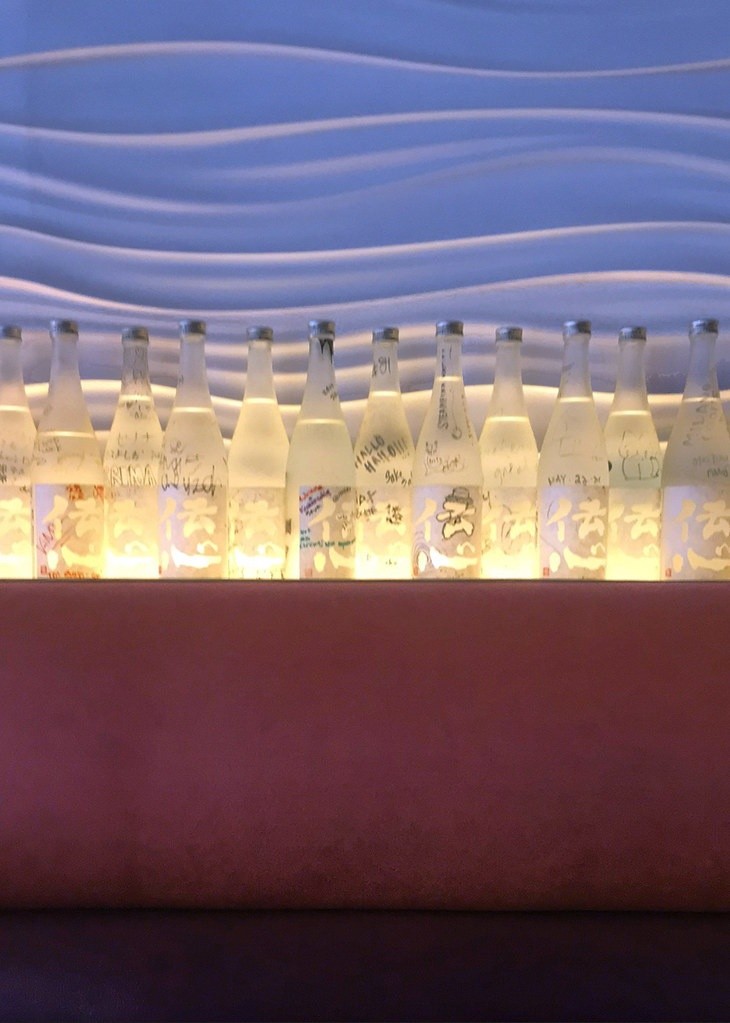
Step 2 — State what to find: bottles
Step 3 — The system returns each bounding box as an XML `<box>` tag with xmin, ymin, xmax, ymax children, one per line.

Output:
<box><xmin>537</xmin><ymin>318</ymin><xmax>611</xmax><ymax>580</ymax></box>
<box><xmin>607</xmin><ymin>326</ymin><xmax>668</xmax><ymax>584</ymax></box>
<box><xmin>352</xmin><ymin>327</ymin><xmax>416</xmax><ymax>582</ymax></box>
<box><xmin>661</xmin><ymin>316</ymin><xmax>730</xmax><ymax>585</ymax></box>
<box><xmin>479</xmin><ymin>325</ymin><xmax>544</xmax><ymax>580</ymax></box>
<box><xmin>225</xmin><ymin>325</ymin><xmax>293</xmax><ymax>581</ymax></box>
<box><xmin>160</xmin><ymin>319</ymin><xmax>228</xmax><ymax>580</ymax></box>
<box><xmin>287</xmin><ymin>320</ymin><xmax>359</xmax><ymax>581</ymax></box>
<box><xmin>413</xmin><ymin>320</ymin><xmax>487</xmax><ymax>582</ymax></box>
<box><xmin>103</xmin><ymin>326</ymin><xmax>168</xmax><ymax>579</ymax></box>
<box><xmin>0</xmin><ymin>324</ymin><xmax>37</xmax><ymax>581</ymax></box>
<box><xmin>35</xmin><ymin>318</ymin><xmax>108</xmax><ymax>579</ymax></box>
